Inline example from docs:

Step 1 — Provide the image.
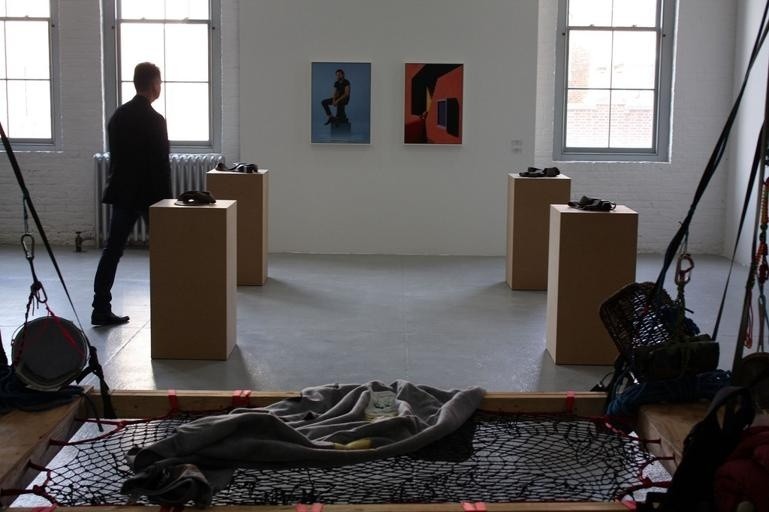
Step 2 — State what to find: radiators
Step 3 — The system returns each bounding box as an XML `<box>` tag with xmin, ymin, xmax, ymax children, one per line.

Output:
<box><xmin>77</xmin><ymin>152</ymin><xmax>224</xmax><ymax>257</ymax></box>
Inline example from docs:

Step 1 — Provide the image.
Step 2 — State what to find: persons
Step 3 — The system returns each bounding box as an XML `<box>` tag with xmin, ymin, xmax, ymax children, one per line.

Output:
<box><xmin>322</xmin><ymin>69</ymin><xmax>351</xmax><ymax>126</ymax></box>
<box><xmin>89</xmin><ymin>62</ymin><xmax>173</xmax><ymax>329</ymax></box>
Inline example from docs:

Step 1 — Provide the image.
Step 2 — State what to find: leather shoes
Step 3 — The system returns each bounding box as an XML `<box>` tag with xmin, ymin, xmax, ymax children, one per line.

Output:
<box><xmin>324</xmin><ymin>115</ymin><xmax>335</xmax><ymax>126</ymax></box>
<box><xmin>90</xmin><ymin>311</ymin><xmax>130</xmax><ymax>326</ymax></box>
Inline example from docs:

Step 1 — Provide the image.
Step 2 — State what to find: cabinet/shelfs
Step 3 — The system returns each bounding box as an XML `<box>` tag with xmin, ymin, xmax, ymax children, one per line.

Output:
<box><xmin>504</xmin><ymin>172</ymin><xmax>572</xmax><ymax>292</ymax></box>
<box><xmin>545</xmin><ymin>202</ymin><xmax>638</xmax><ymax>365</ymax></box>
<box><xmin>205</xmin><ymin>165</ymin><xmax>271</xmax><ymax>286</ymax></box>
<box><xmin>149</xmin><ymin>198</ymin><xmax>236</xmax><ymax>362</ymax></box>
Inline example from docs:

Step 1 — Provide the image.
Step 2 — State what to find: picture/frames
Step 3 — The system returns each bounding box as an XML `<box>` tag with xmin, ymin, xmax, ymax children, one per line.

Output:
<box><xmin>311</xmin><ymin>61</ymin><xmax>372</xmax><ymax>143</ymax></box>
<box><xmin>404</xmin><ymin>64</ymin><xmax>462</xmax><ymax>146</ymax></box>
